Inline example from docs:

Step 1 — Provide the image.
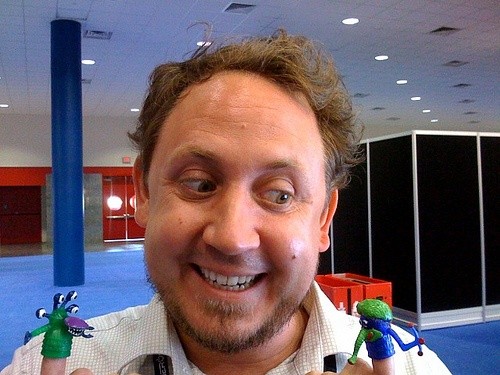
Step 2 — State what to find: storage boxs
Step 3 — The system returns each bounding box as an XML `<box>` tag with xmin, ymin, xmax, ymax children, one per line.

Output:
<box><xmin>315</xmin><ymin>272</ymin><xmax>393</xmax><ymax>316</ymax></box>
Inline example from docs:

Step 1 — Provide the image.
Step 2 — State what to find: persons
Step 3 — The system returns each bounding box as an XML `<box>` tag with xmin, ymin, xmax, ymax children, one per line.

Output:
<box><xmin>0</xmin><ymin>32</ymin><xmax>454</xmax><ymax>375</ymax></box>
